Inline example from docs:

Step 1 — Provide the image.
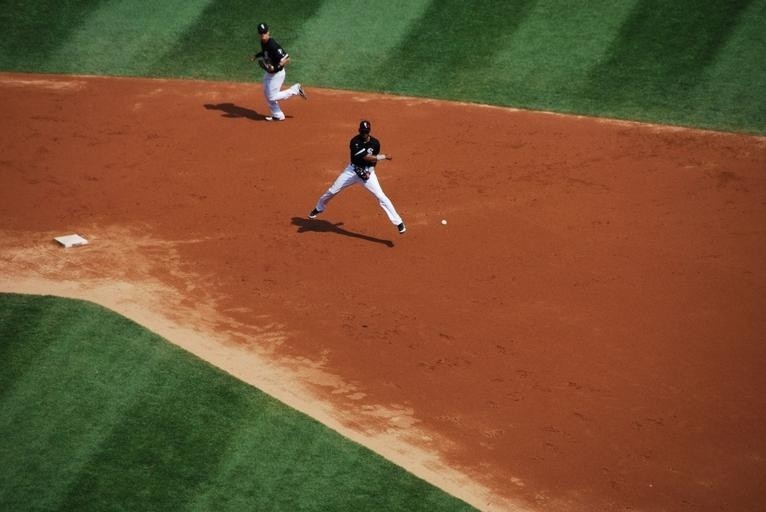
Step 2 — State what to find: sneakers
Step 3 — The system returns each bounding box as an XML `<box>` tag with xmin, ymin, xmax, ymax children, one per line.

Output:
<box><xmin>296</xmin><ymin>83</ymin><xmax>308</xmax><ymax>99</ymax></box>
<box><xmin>397</xmin><ymin>222</ymin><xmax>406</xmax><ymax>234</ymax></box>
<box><xmin>264</xmin><ymin>115</ymin><xmax>286</xmax><ymax>121</ymax></box>
<box><xmin>308</xmin><ymin>207</ymin><xmax>326</xmax><ymax>219</ymax></box>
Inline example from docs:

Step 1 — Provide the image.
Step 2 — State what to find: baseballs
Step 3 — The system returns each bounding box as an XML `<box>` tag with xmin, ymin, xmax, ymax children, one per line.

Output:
<box><xmin>442</xmin><ymin>219</ymin><xmax>447</xmax><ymax>225</ymax></box>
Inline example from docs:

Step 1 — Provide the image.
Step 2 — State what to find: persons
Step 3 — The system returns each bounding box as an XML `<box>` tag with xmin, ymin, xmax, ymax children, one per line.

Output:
<box><xmin>246</xmin><ymin>22</ymin><xmax>307</xmax><ymax>121</ymax></box>
<box><xmin>307</xmin><ymin>120</ymin><xmax>407</xmax><ymax>234</ymax></box>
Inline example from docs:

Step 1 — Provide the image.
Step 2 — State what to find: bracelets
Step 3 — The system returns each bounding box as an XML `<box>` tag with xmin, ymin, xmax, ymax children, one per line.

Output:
<box><xmin>376</xmin><ymin>154</ymin><xmax>386</xmax><ymax>161</ymax></box>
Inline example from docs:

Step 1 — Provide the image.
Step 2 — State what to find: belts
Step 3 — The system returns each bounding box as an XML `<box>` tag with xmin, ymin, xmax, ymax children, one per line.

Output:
<box><xmin>350</xmin><ymin>163</ymin><xmax>371</xmax><ymax>170</ymax></box>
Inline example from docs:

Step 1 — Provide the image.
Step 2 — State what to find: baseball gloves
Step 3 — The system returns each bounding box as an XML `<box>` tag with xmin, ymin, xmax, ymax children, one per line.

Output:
<box><xmin>353</xmin><ymin>166</ymin><xmax>370</xmax><ymax>180</ymax></box>
<box><xmin>259</xmin><ymin>59</ymin><xmax>274</xmax><ymax>73</ymax></box>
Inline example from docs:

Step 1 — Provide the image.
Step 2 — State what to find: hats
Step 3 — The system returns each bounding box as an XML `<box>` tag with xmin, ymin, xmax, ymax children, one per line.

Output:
<box><xmin>359</xmin><ymin>121</ymin><xmax>371</xmax><ymax>132</ymax></box>
<box><xmin>258</xmin><ymin>23</ymin><xmax>268</xmax><ymax>33</ymax></box>
